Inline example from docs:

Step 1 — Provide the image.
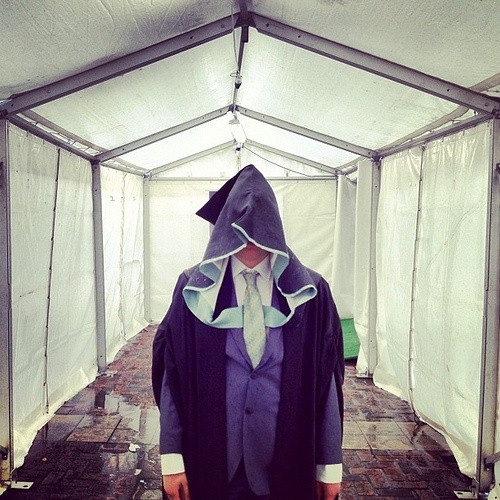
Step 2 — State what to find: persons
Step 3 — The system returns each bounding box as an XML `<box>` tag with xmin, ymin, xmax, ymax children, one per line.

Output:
<box><xmin>146</xmin><ymin>164</ymin><xmax>351</xmax><ymax>500</ymax></box>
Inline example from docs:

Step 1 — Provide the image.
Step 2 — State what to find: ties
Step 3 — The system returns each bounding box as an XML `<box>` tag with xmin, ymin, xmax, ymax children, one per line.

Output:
<box><xmin>241</xmin><ymin>271</ymin><xmax>267</xmax><ymax>370</ymax></box>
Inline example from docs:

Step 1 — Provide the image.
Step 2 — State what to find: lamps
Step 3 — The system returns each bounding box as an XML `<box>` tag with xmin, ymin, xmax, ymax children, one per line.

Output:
<box><xmin>228</xmin><ymin>120</ymin><xmax>246</xmax><ymax>143</ymax></box>
